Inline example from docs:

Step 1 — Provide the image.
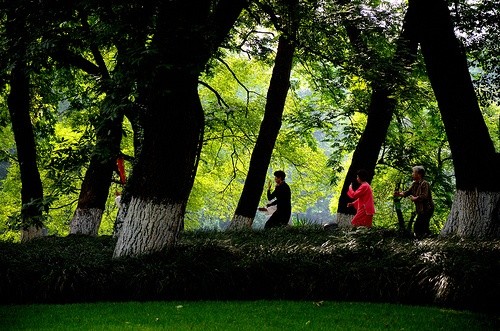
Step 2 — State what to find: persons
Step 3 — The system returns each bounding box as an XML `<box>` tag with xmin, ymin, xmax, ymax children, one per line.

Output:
<box><xmin>394</xmin><ymin>166</ymin><xmax>435</xmax><ymax>240</ymax></box>
<box><xmin>264</xmin><ymin>170</ymin><xmax>292</xmax><ymax>229</ymax></box>
<box><xmin>347</xmin><ymin>172</ymin><xmax>375</xmax><ymax>227</ymax></box>
